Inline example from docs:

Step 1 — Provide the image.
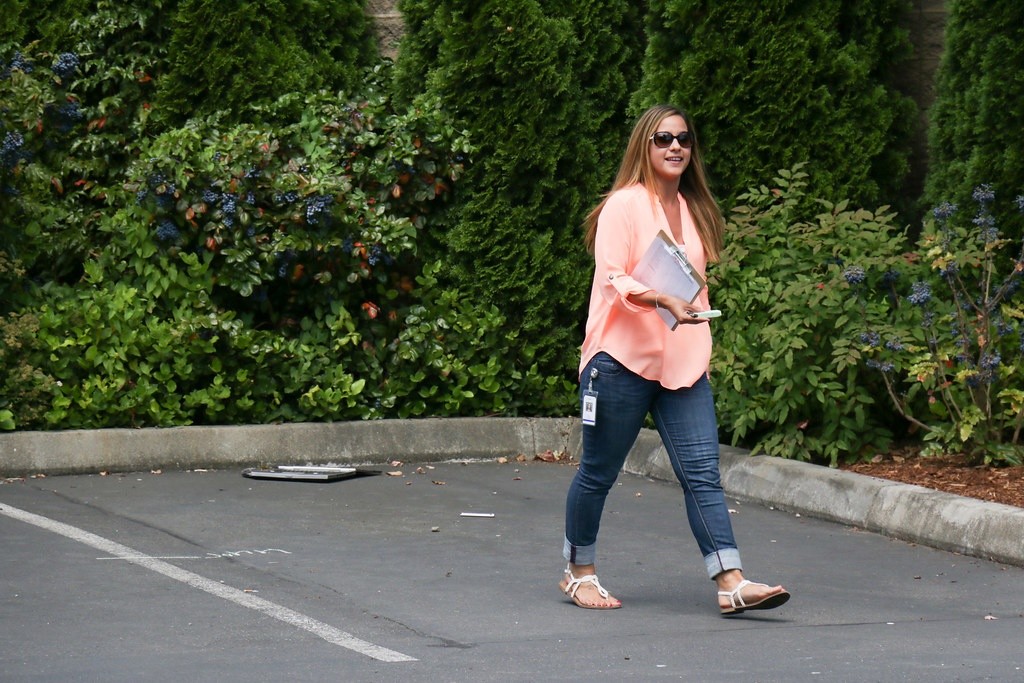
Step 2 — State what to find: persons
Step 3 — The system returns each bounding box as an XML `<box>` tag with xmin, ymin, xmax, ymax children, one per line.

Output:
<box><xmin>560</xmin><ymin>105</ymin><xmax>792</xmax><ymax>617</ymax></box>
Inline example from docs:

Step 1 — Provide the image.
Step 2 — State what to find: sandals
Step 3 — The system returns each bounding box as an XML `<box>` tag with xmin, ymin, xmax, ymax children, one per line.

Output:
<box><xmin>558</xmin><ymin>563</ymin><xmax>620</xmax><ymax>609</ymax></box>
<box><xmin>718</xmin><ymin>580</ymin><xmax>790</xmax><ymax>615</ymax></box>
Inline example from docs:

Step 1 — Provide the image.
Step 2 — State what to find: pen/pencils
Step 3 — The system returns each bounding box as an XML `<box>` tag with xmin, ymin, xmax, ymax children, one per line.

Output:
<box><xmin>691</xmin><ymin>314</ymin><xmax>698</xmax><ymax>317</ymax></box>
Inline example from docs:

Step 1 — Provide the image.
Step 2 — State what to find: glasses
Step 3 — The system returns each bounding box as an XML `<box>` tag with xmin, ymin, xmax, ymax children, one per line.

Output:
<box><xmin>649</xmin><ymin>131</ymin><xmax>694</xmax><ymax>148</ymax></box>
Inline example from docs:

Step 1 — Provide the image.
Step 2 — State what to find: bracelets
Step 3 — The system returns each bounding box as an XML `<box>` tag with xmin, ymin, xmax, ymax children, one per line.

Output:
<box><xmin>656</xmin><ymin>292</ymin><xmax>662</xmax><ymax>309</ymax></box>
